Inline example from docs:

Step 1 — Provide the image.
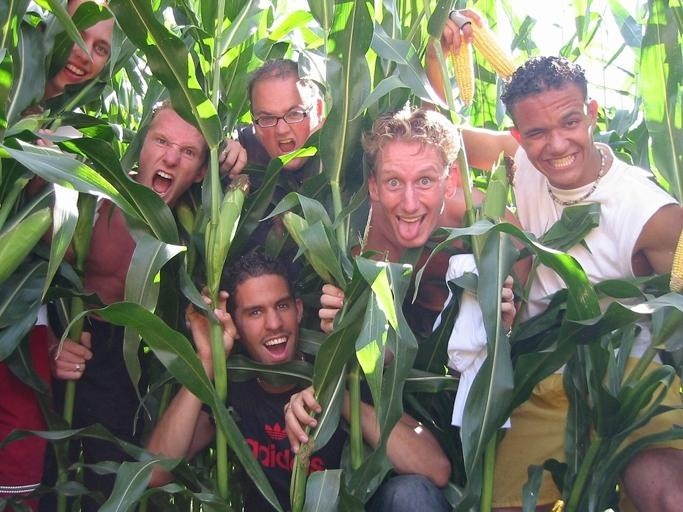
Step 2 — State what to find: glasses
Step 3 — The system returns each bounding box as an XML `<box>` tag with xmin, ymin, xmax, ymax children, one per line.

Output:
<box><xmin>251</xmin><ymin>104</ymin><xmax>314</xmax><ymax>129</ymax></box>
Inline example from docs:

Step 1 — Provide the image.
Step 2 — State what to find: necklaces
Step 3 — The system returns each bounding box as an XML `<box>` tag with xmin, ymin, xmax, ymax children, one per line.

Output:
<box><xmin>546</xmin><ymin>146</ymin><xmax>605</xmax><ymax>205</ymax></box>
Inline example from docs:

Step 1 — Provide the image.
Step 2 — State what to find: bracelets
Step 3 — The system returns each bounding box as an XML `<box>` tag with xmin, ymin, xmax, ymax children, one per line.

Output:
<box><xmin>506</xmin><ymin>327</ymin><xmax>511</xmax><ymax>337</ymax></box>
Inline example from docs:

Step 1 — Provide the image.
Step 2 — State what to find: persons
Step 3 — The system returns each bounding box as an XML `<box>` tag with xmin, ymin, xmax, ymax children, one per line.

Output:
<box><xmin>0</xmin><ymin>0</ymin><xmax>115</xmax><ymax>512</ymax></box>
<box><xmin>48</xmin><ymin>99</ymin><xmax>211</xmax><ymax>491</ymax></box>
<box><xmin>426</xmin><ymin>9</ymin><xmax>682</xmax><ymax>511</ymax></box>
<box><xmin>318</xmin><ymin>108</ymin><xmax>534</xmax><ymax>366</ymax></box>
<box><xmin>146</xmin><ymin>255</ymin><xmax>451</xmax><ymax>512</ymax></box>
<box><xmin>219</xmin><ymin>62</ymin><xmax>326</xmax><ymax>275</ymax></box>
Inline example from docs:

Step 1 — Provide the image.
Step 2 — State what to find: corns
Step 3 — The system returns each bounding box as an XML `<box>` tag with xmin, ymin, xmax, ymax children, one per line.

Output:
<box><xmin>7</xmin><ymin>105</ymin><xmax>49</xmax><ymax>138</ymax></box>
<box><xmin>214</xmin><ymin>175</ymin><xmax>248</xmax><ymax>272</ymax></box>
<box><xmin>448</xmin><ymin>32</ymin><xmax>475</xmax><ymax>109</ymax></box>
<box><xmin>669</xmin><ymin>229</ymin><xmax>683</xmax><ymax>290</ymax></box>
<box><xmin>467</xmin><ymin>18</ymin><xmax>516</xmax><ymax>80</ymax></box>
<box><xmin>479</xmin><ymin>155</ymin><xmax>512</xmax><ymax>223</ymax></box>
<box><xmin>0</xmin><ymin>202</ymin><xmax>53</xmax><ymax>283</ymax></box>
<box><xmin>280</xmin><ymin>209</ymin><xmax>333</xmax><ymax>280</ymax></box>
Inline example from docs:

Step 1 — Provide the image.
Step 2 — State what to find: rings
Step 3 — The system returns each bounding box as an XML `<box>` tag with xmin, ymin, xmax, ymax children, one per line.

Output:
<box><xmin>73</xmin><ymin>364</ymin><xmax>80</xmax><ymax>372</ymax></box>
<box><xmin>286</xmin><ymin>406</ymin><xmax>290</xmax><ymax>412</ymax></box>
<box><xmin>222</xmin><ymin>150</ymin><xmax>228</xmax><ymax>154</ymax></box>
